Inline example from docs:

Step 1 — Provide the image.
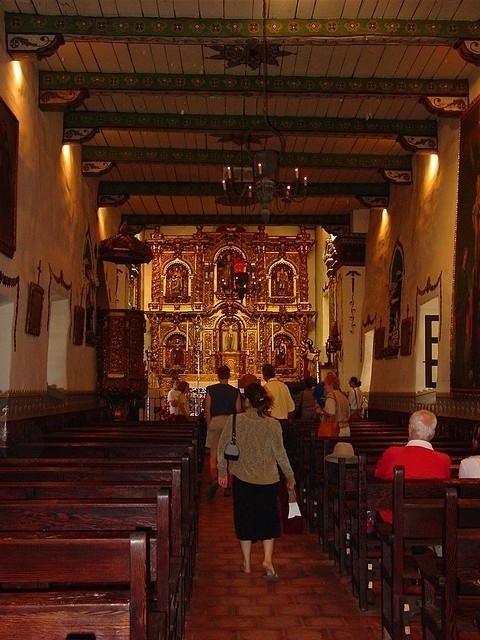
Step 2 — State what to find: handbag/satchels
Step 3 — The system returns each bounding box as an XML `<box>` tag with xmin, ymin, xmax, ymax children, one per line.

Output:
<box><xmin>317</xmin><ymin>419</ymin><xmax>340</xmax><ymax>438</ymax></box>
<box><xmin>224</xmin><ymin>440</ymin><xmax>240</xmax><ymax>461</ymax></box>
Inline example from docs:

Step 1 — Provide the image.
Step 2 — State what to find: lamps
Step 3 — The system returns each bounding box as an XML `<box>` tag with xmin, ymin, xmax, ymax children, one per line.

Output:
<box><xmin>215</xmin><ymin>150</ymin><xmax>317</xmax><ymax>225</ymax></box>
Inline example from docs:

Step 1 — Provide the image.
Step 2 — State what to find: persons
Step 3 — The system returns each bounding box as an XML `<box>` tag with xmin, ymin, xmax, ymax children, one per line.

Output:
<box><xmin>275</xmin><ymin>268</ymin><xmax>290</xmax><ymax>294</ymax></box>
<box><xmin>167</xmin><ymin>377</ymin><xmax>180</xmax><ymax>415</ymax></box>
<box><xmin>221</xmin><ymin>253</ymin><xmax>235</xmax><ymax>289</ymax></box>
<box><xmin>222</xmin><ymin>317</ymin><xmax>242</xmax><ymax>351</ymax></box>
<box><xmin>372</xmin><ymin>409</ymin><xmax>453</xmax><ymax>524</ymax></box>
<box><xmin>278</xmin><ymin>337</ymin><xmax>287</xmax><ymax>367</ymax></box>
<box><xmin>203</xmin><ymin>365</ymin><xmax>242</xmax><ymax>500</ymax></box>
<box><xmin>165</xmin><ymin>337</ymin><xmax>187</xmax><ymax>368</ymax></box>
<box><xmin>458</xmin><ymin>422</ymin><xmax>480</xmax><ymax>480</ymax></box>
<box><xmin>217</xmin><ymin>382</ymin><xmax>297</xmax><ymax>580</ymax></box>
<box><xmin>314</xmin><ymin>374</ymin><xmax>351</xmax><ymax>438</ymax></box>
<box><xmin>276</xmin><ymin>368</ymin><xmax>365</xmax><ymax>495</ymax></box>
<box><xmin>167</xmin><ymin>265</ymin><xmax>186</xmax><ymax>296</ymax></box>
<box><xmin>261</xmin><ymin>363</ymin><xmax>296</xmax><ymax>450</ymax></box>
<box><xmin>176</xmin><ymin>380</ymin><xmax>197</xmax><ymax>421</ymax></box>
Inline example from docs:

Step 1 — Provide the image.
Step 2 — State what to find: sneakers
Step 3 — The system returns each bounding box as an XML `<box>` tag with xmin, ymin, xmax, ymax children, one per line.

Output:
<box><xmin>207</xmin><ymin>481</ymin><xmax>217</xmax><ymax>498</ymax></box>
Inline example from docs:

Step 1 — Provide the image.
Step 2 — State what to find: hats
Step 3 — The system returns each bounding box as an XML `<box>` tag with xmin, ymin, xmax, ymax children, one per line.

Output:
<box><xmin>325</xmin><ymin>442</ymin><xmax>361</xmax><ymax>464</ymax></box>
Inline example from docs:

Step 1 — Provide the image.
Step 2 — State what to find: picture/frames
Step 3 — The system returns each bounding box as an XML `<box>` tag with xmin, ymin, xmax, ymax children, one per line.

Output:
<box><xmin>25</xmin><ymin>281</ymin><xmax>44</xmax><ymax>337</ymax></box>
<box><xmin>0</xmin><ymin>94</ymin><xmax>19</xmax><ymax>259</ymax></box>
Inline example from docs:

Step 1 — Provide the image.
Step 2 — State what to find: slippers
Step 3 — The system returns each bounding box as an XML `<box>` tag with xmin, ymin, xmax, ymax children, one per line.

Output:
<box><xmin>261</xmin><ymin>572</ymin><xmax>279</xmax><ymax>581</ymax></box>
<box><xmin>240</xmin><ymin>564</ymin><xmax>248</xmax><ymax>576</ymax></box>
